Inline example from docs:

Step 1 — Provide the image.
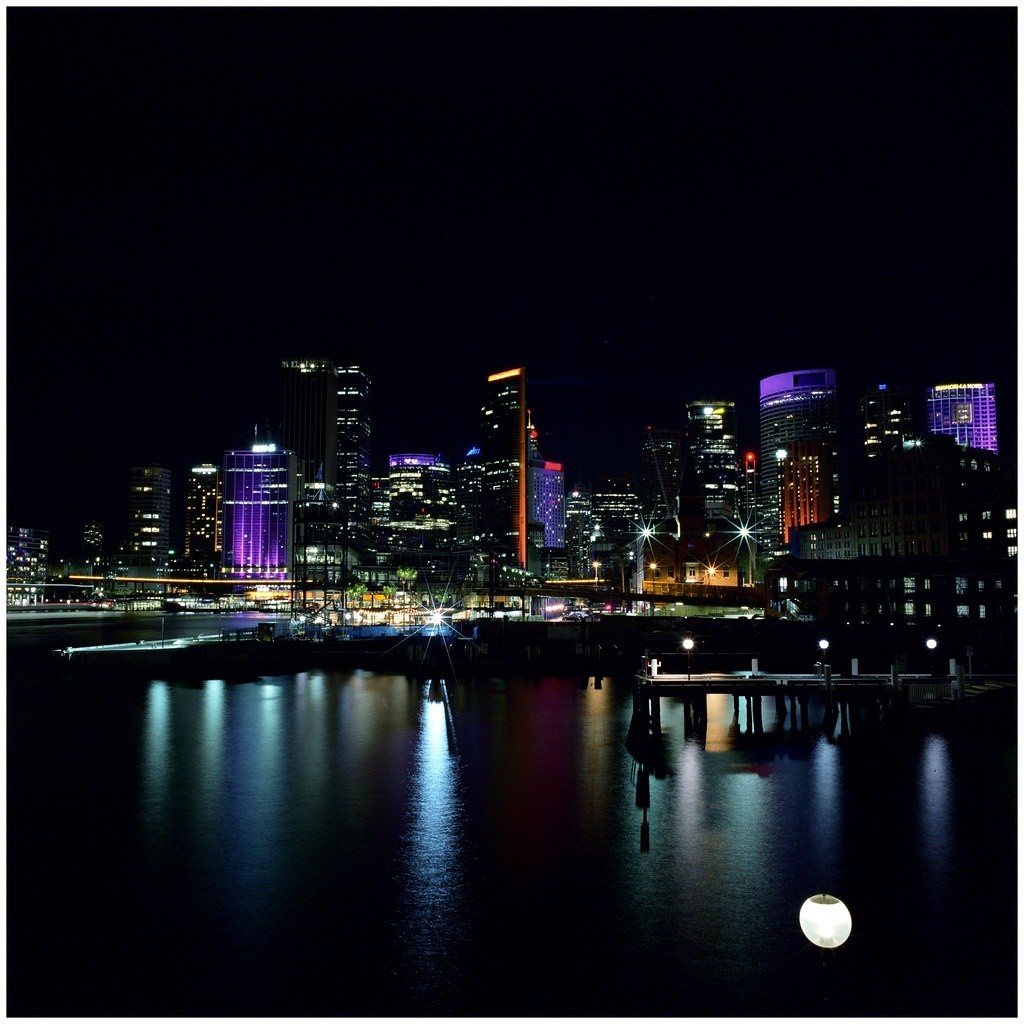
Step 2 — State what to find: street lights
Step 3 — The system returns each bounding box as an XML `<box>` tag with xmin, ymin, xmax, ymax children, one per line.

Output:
<box><xmin>745</xmin><ymin>450</ymin><xmax>756</xmax><ymax>549</ymax></box>
<box><xmin>925</xmin><ymin>639</ymin><xmax>940</xmax><ymax>677</ymax></box>
<box><xmin>684</xmin><ymin>639</ymin><xmax>696</xmax><ymax>682</ymax></box>
<box><xmin>820</xmin><ymin>639</ymin><xmax>830</xmax><ymax>668</ymax></box>
